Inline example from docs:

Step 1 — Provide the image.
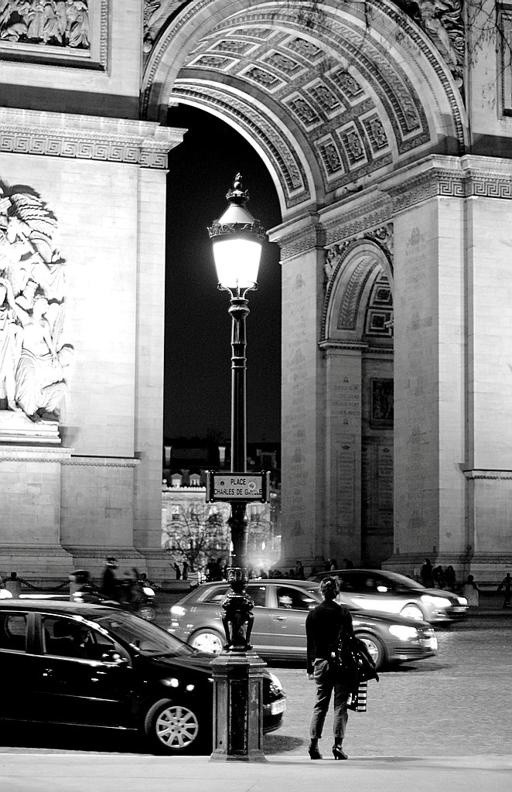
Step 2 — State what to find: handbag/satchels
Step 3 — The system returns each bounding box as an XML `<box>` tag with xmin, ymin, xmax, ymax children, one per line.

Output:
<box><xmin>345</xmin><ymin>680</ymin><xmax>368</xmax><ymax>713</ymax></box>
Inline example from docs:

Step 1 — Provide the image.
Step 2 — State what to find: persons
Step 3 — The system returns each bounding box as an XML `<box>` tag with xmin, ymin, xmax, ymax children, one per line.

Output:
<box><xmin>446</xmin><ymin>564</ymin><xmax>460</xmax><ymax>589</ymax></box>
<box><xmin>495</xmin><ymin>570</ymin><xmax>512</xmax><ymax>610</ymax></box>
<box><xmin>64</xmin><ymin>622</ymin><xmax>104</xmax><ymax>661</ymax></box>
<box><xmin>99</xmin><ymin>554</ymin><xmax>126</xmax><ymax>601</ymax></box>
<box><xmin>303</xmin><ymin>573</ymin><xmax>358</xmax><ymax>761</ymax></box>
<box><xmin>433</xmin><ymin>565</ymin><xmax>446</xmax><ymax>588</ymax></box>
<box><xmin>461</xmin><ymin>574</ymin><xmax>483</xmax><ymax>599</ymax></box>
<box><xmin>172</xmin><ymin>554</ymin><xmax>358</xmax><ymax>584</ymax></box>
<box><xmin>419</xmin><ymin>558</ymin><xmax>437</xmax><ymax>589</ymax></box>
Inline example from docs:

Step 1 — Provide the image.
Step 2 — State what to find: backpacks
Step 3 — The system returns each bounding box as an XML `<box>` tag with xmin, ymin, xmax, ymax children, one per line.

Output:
<box><xmin>335</xmin><ymin>625</ymin><xmax>379</xmax><ymax>687</ymax></box>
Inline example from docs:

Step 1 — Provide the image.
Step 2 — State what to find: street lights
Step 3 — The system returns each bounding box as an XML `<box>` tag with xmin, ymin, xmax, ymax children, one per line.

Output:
<box><xmin>205</xmin><ymin>171</ymin><xmax>271</xmax><ymax>763</ymax></box>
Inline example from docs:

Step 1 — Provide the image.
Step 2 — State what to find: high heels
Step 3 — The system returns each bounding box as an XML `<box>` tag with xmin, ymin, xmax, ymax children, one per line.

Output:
<box><xmin>310</xmin><ymin>743</ymin><xmax>349</xmax><ymax>760</ymax></box>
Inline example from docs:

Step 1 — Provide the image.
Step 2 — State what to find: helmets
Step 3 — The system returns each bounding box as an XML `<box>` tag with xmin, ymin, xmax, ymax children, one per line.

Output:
<box><xmin>105</xmin><ymin>556</ymin><xmax>119</xmax><ymax>570</ymax></box>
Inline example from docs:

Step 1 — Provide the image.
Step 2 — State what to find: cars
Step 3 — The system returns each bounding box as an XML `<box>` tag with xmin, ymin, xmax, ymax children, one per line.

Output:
<box><xmin>1</xmin><ymin>597</ymin><xmax>286</xmax><ymax>756</ymax></box>
<box><xmin>301</xmin><ymin>567</ymin><xmax>470</xmax><ymax>628</ymax></box>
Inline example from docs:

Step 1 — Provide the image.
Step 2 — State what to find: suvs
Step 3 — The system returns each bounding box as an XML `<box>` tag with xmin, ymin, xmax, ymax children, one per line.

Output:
<box><xmin>165</xmin><ymin>577</ymin><xmax>440</xmax><ymax>680</ymax></box>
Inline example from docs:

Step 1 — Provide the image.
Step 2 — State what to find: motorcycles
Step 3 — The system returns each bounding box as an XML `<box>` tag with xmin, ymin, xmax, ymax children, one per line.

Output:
<box><xmin>70</xmin><ymin>567</ymin><xmax>158</xmax><ymax>621</ymax></box>
